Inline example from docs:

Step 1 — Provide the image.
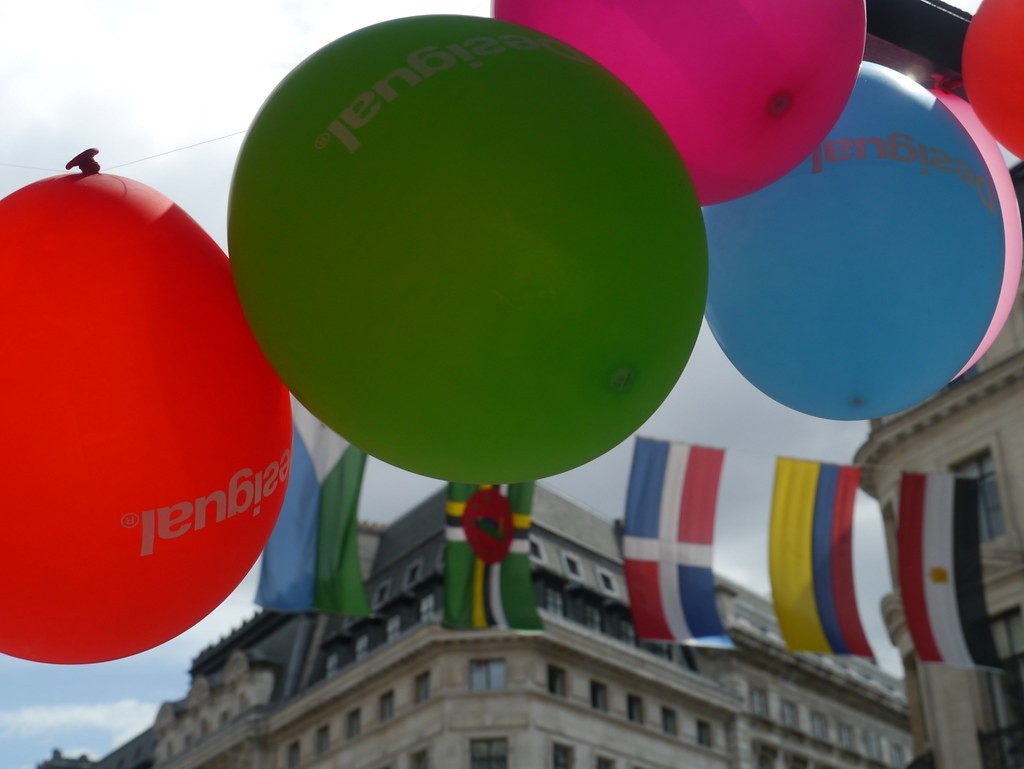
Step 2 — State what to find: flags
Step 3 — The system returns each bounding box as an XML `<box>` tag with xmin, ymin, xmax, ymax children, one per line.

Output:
<box><xmin>766</xmin><ymin>456</ymin><xmax>873</xmax><ymax>662</ymax></box>
<box><xmin>897</xmin><ymin>469</ymin><xmax>1004</xmax><ymax>675</ymax></box>
<box><xmin>440</xmin><ymin>479</ymin><xmax>547</xmax><ymax>635</ymax></box>
<box><xmin>620</xmin><ymin>437</ymin><xmax>736</xmax><ymax>650</ymax></box>
<box><xmin>251</xmin><ymin>393</ymin><xmax>375</xmax><ymax>620</ymax></box>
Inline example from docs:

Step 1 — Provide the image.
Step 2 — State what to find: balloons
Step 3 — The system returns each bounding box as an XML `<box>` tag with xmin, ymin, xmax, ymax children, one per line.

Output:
<box><xmin>1</xmin><ymin>0</ymin><xmax>1024</xmax><ymax>666</ymax></box>
